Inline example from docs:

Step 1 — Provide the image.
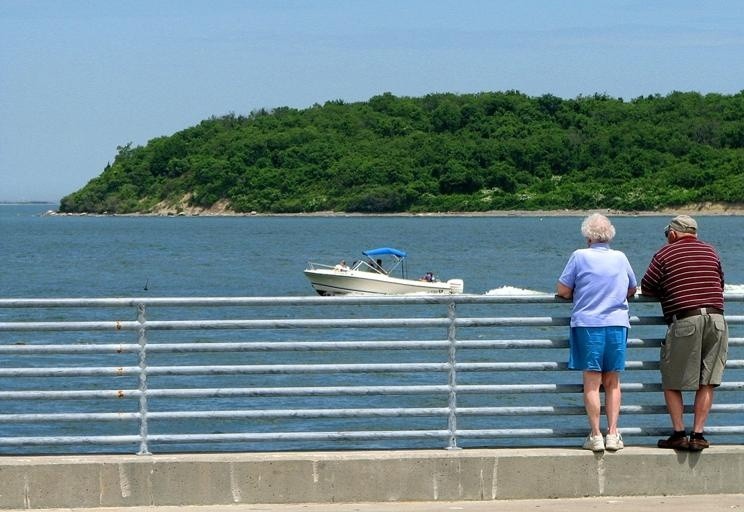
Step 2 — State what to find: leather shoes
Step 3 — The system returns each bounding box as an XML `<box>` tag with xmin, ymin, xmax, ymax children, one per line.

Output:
<box><xmin>689</xmin><ymin>437</ymin><xmax>709</xmax><ymax>451</ymax></box>
<box><xmin>657</xmin><ymin>436</ymin><xmax>691</xmax><ymax>450</ymax></box>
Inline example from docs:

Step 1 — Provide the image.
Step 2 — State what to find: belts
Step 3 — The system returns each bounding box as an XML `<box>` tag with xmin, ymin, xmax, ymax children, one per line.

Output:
<box><xmin>664</xmin><ymin>307</ymin><xmax>723</xmax><ymax>325</ymax></box>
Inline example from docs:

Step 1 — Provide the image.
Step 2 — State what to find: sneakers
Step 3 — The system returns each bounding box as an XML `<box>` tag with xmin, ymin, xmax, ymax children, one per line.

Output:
<box><xmin>605</xmin><ymin>428</ymin><xmax>624</xmax><ymax>450</ymax></box>
<box><xmin>582</xmin><ymin>431</ymin><xmax>605</xmax><ymax>451</ymax></box>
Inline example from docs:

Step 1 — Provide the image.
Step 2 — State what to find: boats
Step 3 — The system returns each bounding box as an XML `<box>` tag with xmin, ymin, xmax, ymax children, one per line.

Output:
<box><xmin>302</xmin><ymin>245</ymin><xmax>466</xmax><ymax>298</ymax></box>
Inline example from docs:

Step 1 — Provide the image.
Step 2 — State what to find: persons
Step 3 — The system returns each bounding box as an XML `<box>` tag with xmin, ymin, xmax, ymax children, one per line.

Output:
<box><xmin>353</xmin><ymin>262</ymin><xmax>360</xmax><ymax>271</ymax></box>
<box><xmin>556</xmin><ymin>212</ymin><xmax>638</xmax><ymax>452</ymax></box>
<box><xmin>368</xmin><ymin>264</ymin><xmax>374</xmax><ymax>272</ymax></box>
<box><xmin>334</xmin><ymin>259</ymin><xmax>350</xmax><ymax>271</ymax></box>
<box><xmin>376</xmin><ymin>259</ymin><xmax>384</xmax><ymax>273</ymax></box>
<box><xmin>641</xmin><ymin>213</ymin><xmax>731</xmax><ymax>451</ymax></box>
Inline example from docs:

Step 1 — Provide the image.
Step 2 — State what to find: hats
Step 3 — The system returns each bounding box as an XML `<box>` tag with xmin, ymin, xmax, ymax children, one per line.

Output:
<box><xmin>663</xmin><ymin>214</ymin><xmax>697</xmax><ymax>234</ymax></box>
